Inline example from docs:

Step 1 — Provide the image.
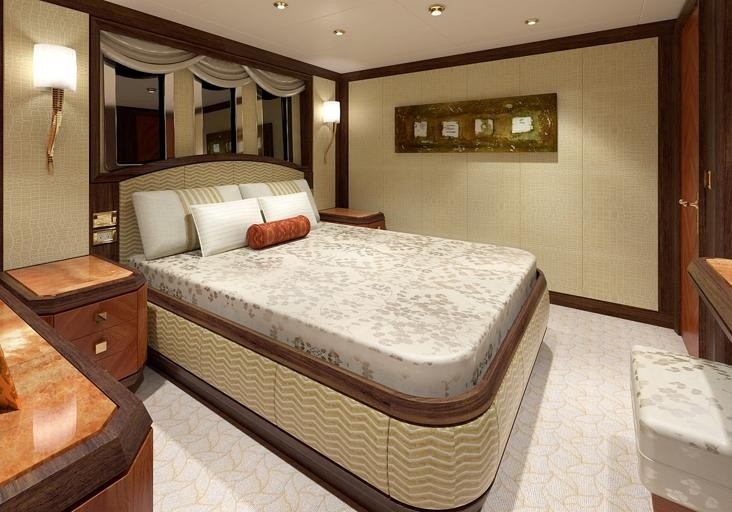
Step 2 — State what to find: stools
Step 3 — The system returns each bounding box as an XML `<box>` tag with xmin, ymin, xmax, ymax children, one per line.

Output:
<box><xmin>630</xmin><ymin>338</ymin><xmax>732</xmax><ymax>512</ymax></box>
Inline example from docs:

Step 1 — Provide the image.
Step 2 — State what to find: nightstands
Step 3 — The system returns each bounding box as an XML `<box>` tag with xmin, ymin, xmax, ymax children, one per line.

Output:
<box><xmin>2</xmin><ymin>253</ymin><xmax>148</xmax><ymax>393</ymax></box>
<box><xmin>319</xmin><ymin>208</ymin><xmax>385</xmax><ymax>229</ymax></box>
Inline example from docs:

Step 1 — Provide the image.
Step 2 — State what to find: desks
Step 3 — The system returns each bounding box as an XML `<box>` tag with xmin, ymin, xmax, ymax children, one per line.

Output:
<box><xmin>2</xmin><ymin>284</ymin><xmax>152</xmax><ymax>512</ymax></box>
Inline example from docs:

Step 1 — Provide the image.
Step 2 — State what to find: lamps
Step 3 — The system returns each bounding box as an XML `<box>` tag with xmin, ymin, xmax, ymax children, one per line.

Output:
<box><xmin>32</xmin><ymin>44</ymin><xmax>78</xmax><ymax>165</ymax></box>
<box><xmin>322</xmin><ymin>100</ymin><xmax>341</xmax><ymax>164</ymax></box>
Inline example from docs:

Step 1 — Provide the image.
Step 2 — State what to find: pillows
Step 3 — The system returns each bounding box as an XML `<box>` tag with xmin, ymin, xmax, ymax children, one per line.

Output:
<box><xmin>130</xmin><ymin>178</ymin><xmax>322</xmax><ymax>262</ymax></box>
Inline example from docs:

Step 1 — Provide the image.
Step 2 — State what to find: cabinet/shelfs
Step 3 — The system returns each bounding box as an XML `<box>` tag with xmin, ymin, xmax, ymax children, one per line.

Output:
<box><xmin>206</xmin><ymin>123</ymin><xmax>273</xmax><ymax>157</ymax></box>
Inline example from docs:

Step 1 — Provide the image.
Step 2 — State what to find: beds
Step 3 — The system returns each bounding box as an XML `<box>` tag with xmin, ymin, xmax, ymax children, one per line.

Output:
<box><xmin>119</xmin><ymin>153</ymin><xmax>554</xmax><ymax>510</ymax></box>
